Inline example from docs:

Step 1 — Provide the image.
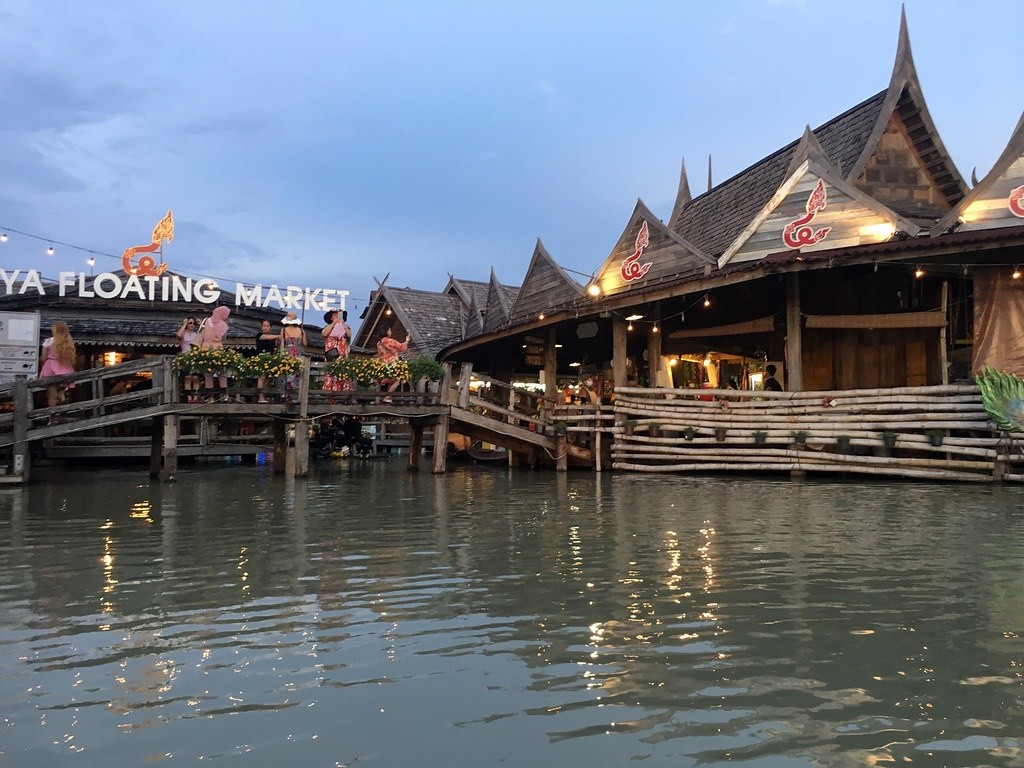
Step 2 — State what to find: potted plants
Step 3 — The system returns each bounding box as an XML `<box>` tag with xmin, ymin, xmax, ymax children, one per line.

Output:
<box><xmin>926</xmin><ymin>428</ymin><xmax>946</xmax><ymax>446</ymax></box>
<box><xmin>836</xmin><ymin>433</ymin><xmax>851</xmax><ymax>449</ymax></box>
<box><xmin>753</xmin><ymin>430</ymin><xmax>768</xmax><ymax>445</ymax></box>
<box><xmin>647</xmin><ymin>422</ymin><xmax>660</xmax><ymax>436</ymax></box>
<box><xmin>682</xmin><ymin>426</ymin><xmax>699</xmax><ymax>441</ymax></box>
<box><xmin>795</xmin><ymin>431</ymin><xmax>812</xmax><ymax>444</ymax></box>
<box><xmin>713</xmin><ymin>427</ymin><xmax>728</xmax><ymax>442</ymax></box>
<box><xmin>555</xmin><ymin>422</ymin><xmax>569</xmax><ymax>437</ymax></box>
<box><xmin>621</xmin><ymin>419</ymin><xmax>637</xmax><ymax>435</ymax></box>
<box><xmin>881</xmin><ymin>431</ymin><xmax>900</xmax><ymax>447</ymax></box>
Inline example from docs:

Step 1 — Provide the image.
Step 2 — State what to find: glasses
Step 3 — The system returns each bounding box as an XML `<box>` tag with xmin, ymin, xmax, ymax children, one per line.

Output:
<box><xmin>188</xmin><ymin>322</ymin><xmax>196</xmax><ymax>325</ymax></box>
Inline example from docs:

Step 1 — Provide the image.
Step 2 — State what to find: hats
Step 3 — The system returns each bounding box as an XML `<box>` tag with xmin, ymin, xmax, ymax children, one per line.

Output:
<box><xmin>324</xmin><ymin>308</ymin><xmax>348</xmax><ymax>324</ymax></box>
<box><xmin>280</xmin><ymin>312</ymin><xmax>302</xmax><ymax>325</ymax></box>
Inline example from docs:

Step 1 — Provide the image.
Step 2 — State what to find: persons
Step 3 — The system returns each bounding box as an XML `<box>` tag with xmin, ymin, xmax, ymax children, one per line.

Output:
<box><xmin>203</xmin><ymin>305</ymin><xmax>231</xmax><ymax>404</ymax></box>
<box><xmin>374</xmin><ymin>324</ymin><xmax>411</xmax><ymax>404</ymax></box>
<box><xmin>627</xmin><ymin>374</ymin><xmax>648</xmax><ymax>398</ymax></box>
<box><xmin>760</xmin><ymin>363</ymin><xmax>782</xmax><ymax>402</ymax></box>
<box><xmin>40</xmin><ymin>320</ymin><xmax>77</xmax><ymax>407</ymax></box>
<box><xmin>255</xmin><ymin>319</ymin><xmax>281</xmax><ymax>404</ymax></box>
<box><xmin>719</xmin><ymin>379</ymin><xmax>740</xmax><ymax>402</ymax></box>
<box><xmin>566</xmin><ymin>395</ymin><xmax>581</xmax><ymax>443</ymax></box>
<box><xmin>517</xmin><ymin>387</ymin><xmax>543</xmax><ymax>429</ymax></box>
<box><xmin>280</xmin><ymin>312</ymin><xmax>308</xmax><ymax>403</ymax></box>
<box><xmin>176</xmin><ymin>317</ymin><xmax>204</xmax><ymax>403</ymax></box>
<box><xmin>321</xmin><ymin>309</ymin><xmax>358</xmax><ymax>406</ymax></box>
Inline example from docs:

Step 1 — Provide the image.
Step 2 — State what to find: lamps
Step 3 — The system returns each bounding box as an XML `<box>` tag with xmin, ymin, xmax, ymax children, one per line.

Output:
<box><xmin>894</xmin><ymin>230</ymin><xmax>907</xmax><ymax>241</ymax></box>
<box><xmin>948</xmin><ymin>216</ymin><xmax>966</xmax><ymax>233</ymax></box>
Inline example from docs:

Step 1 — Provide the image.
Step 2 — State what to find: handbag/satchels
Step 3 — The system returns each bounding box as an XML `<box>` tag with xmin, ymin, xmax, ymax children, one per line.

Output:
<box><xmin>325</xmin><ymin>349</ymin><xmax>339</xmax><ymax>361</ymax></box>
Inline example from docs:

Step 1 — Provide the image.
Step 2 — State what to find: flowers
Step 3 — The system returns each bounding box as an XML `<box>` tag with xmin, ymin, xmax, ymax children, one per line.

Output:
<box><xmin>326</xmin><ymin>357</ymin><xmax>410</xmax><ymax>384</ymax></box>
<box><xmin>171</xmin><ymin>345</ymin><xmax>305</xmax><ymax>379</ymax></box>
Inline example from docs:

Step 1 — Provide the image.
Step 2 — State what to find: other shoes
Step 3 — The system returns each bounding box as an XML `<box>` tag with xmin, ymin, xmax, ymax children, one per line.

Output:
<box><xmin>382</xmin><ymin>399</ymin><xmax>392</xmax><ymax>402</ymax></box>
<box><xmin>188</xmin><ymin>400</ymin><xmax>198</xmax><ymax>403</ymax></box>
<box><xmin>258</xmin><ymin>400</ymin><xmax>268</xmax><ymax>403</ymax></box>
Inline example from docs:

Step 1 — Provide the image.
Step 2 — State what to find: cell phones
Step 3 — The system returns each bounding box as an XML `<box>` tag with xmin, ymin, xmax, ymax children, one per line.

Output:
<box><xmin>406</xmin><ymin>329</ymin><xmax>411</xmax><ymax>336</ymax></box>
<box><xmin>338</xmin><ymin>310</ymin><xmax>344</xmax><ymax>320</ymax></box>
